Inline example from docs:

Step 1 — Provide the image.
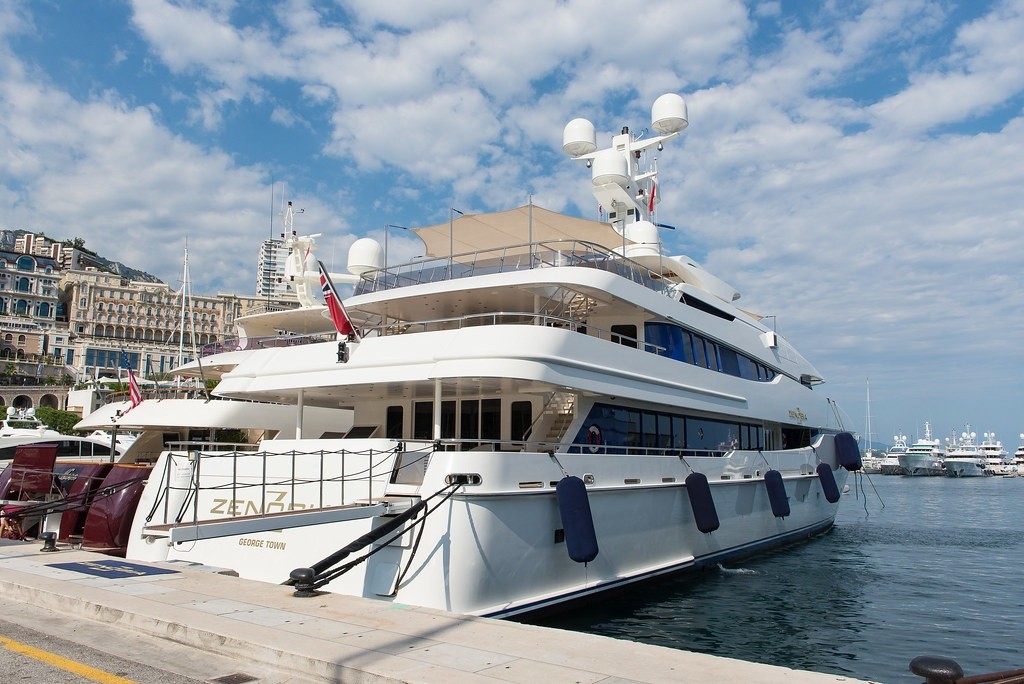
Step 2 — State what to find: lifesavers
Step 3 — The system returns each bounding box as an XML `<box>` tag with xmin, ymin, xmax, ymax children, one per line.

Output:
<box><xmin>587</xmin><ymin>426</ymin><xmax>601</xmax><ymax>453</ymax></box>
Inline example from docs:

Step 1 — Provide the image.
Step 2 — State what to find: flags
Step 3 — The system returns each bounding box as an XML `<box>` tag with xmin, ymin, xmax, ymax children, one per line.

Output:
<box><xmin>650</xmin><ymin>183</ymin><xmax>656</xmax><ymax>212</ymax></box>
<box><xmin>318</xmin><ymin>261</ymin><xmax>353</xmax><ymax>335</ymax></box>
<box><xmin>121</xmin><ymin>349</ymin><xmax>144</xmax><ymax>408</ymax></box>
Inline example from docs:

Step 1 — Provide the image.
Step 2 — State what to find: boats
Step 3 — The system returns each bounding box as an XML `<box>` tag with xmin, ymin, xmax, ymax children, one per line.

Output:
<box><xmin>74</xmin><ymin>90</ymin><xmax>861</xmax><ymax>621</ymax></box>
<box><xmin>0</xmin><ymin>236</ymin><xmax>283</xmax><ymax>559</ymax></box>
<box><xmin>852</xmin><ymin>377</ymin><xmax>1024</xmax><ymax>479</ymax></box>
